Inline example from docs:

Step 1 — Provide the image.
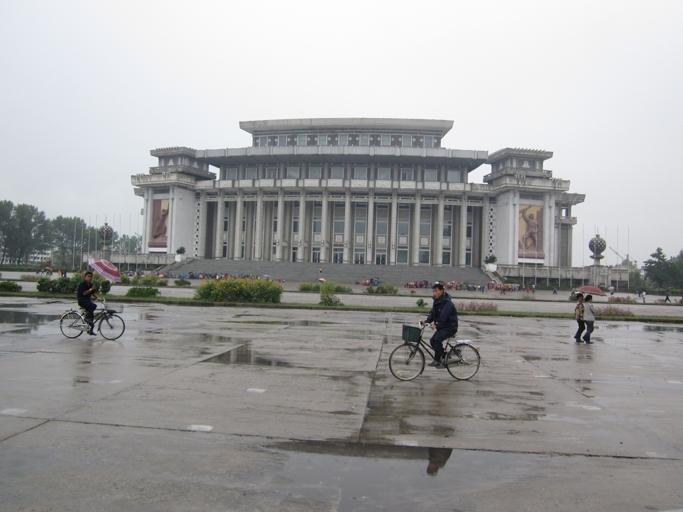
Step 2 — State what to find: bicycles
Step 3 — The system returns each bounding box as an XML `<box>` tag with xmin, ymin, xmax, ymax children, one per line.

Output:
<box><xmin>387</xmin><ymin>320</ymin><xmax>482</xmax><ymax>382</ymax></box>
<box><xmin>59</xmin><ymin>290</ymin><xmax>124</xmax><ymax>341</ymax></box>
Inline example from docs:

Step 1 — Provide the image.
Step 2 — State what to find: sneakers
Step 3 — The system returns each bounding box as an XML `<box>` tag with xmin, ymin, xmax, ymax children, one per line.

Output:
<box><xmin>84</xmin><ymin>318</ymin><xmax>93</xmax><ymax>324</ymax></box>
<box><xmin>87</xmin><ymin>332</ymin><xmax>96</xmax><ymax>336</ymax></box>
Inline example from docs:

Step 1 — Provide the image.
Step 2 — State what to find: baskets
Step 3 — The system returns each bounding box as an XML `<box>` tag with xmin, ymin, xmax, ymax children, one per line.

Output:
<box><xmin>401</xmin><ymin>324</ymin><xmax>422</xmax><ymax>343</ymax></box>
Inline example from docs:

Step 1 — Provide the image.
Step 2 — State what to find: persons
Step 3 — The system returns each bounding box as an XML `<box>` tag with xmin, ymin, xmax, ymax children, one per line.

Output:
<box><xmin>420</xmin><ymin>283</ymin><xmax>458</xmax><ymax>366</ymax></box>
<box><xmin>665</xmin><ymin>288</ymin><xmax>671</xmax><ymax>302</ymax></box>
<box><xmin>481</xmin><ymin>282</ymin><xmax>557</xmax><ymax>295</ymax></box>
<box><xmin>77</xmin><ymin>270</ymin><xmax>97</xmax><ymax>335</ymax></box>
<box><xmin>641</xmin><ymin>288</ymin><xmax>647</xmax><ymax>303</ymax></box>
<box><xmin>582</xmin><ymin>294</ymin><xmax>596</xmax><ymax>344</ymax></box>
<box><xmin>573</xmin><ymin>293</ymin><xmax>586</xmax><ymax>343</ymax></box>
<box><xmin>519</xmin><ymin>205</ymin><xmax>539</xmax><ymax>251</ymax></box>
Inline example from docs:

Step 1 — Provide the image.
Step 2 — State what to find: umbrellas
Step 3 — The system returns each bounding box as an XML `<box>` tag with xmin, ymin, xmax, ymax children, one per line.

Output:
<box><xmin>87</xmin><ymin>257</ymin><xmax>122</xmax><ymax>289</ymax></box>
<box><xmin>575</xmin><ymin>286</ymin><xmax>607</xmax><ymax>298</ymax></box>
